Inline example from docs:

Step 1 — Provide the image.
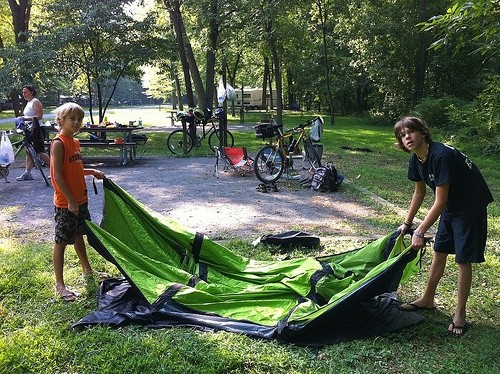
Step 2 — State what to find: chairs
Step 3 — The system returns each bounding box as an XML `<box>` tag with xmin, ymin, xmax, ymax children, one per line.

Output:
<box><xmin>213</xmin><ymin>145</ymin><xmax>254</xmax><ymax>177</ymax></box>
<box><xmin>254</xmin><ymin>120</ymin><xmax>274</xmax><ymax>138</ymax></box>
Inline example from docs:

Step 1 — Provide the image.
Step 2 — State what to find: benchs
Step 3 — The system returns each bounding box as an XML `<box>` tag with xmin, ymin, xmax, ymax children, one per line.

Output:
<box><xmin>168</xmin><ymin>117</ymin><xmax>177</xmax><ymax>119</ymax></box>
<box><xmin>40</xmin><ymin>137</ymin><xmax>146</xmax><ymax>148</ymax></box>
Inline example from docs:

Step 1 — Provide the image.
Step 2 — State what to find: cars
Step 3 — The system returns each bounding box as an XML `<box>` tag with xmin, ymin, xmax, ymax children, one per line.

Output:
<box><xmin>124</xmin><ymin>99</ymin><xmax>141</xmax><ymax>106</ymax></box>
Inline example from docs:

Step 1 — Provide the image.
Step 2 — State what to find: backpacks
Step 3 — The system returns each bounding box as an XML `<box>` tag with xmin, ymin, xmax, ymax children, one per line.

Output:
<box><xmin>311</xmin><ymin>161</ymin><xmax>337</xmax><ymax>192</ymax></box>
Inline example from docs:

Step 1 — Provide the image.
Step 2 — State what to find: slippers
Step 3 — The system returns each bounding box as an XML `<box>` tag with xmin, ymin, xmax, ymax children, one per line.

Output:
<box><xmin>399</xmin><ymin>300</ymin><xmax>436</xmax><ymax>312</ymax></box>
<box><xmin>448</xmin><ymin>322</ymin><xmax>467</xmax><ymax>336</ymax></box>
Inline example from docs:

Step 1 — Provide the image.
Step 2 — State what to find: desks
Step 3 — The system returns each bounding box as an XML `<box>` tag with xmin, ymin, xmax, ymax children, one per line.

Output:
<box><xmin>167</xmin><ymin>110</ymin><xmax>179</xmax><ymax>126</ymax></box>
<box><xmin>42</xmin><ymin>125</ymin><xmax>143</xmax><ymax>168</ymax></box>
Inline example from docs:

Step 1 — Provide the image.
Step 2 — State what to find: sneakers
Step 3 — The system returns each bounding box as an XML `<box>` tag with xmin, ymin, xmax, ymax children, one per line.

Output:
<box><xmin>16</xmin><ymin>171</ymin><xmax>33</xmax><ymax>181</ymax></box>
<box><xmin>48</xmin><ymin>175</ymin><xmax>50</xmax><ymax>179</ymax></box>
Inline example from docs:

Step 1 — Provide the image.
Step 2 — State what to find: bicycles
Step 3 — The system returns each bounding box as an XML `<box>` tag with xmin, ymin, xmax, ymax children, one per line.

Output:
<box><xmin>166</xmin><ymin>107</ymin><xmax>234</xmax><ymax>156</ymax></box>
<box><xmin>253</xmin><ymin>115</ymin><xmax>325</xmax><ymax>184</ymax></box>
<box><xmin>0</xmin><ymin>122</ymin><xmax>51</xmax><ymax>187</ymax></box>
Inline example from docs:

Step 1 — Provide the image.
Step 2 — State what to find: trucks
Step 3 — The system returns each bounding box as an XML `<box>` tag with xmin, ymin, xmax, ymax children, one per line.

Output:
<box><xmin>235</xmin><ymin>89</ymin><xmax>277</xmax><ymax>111</ymax></box>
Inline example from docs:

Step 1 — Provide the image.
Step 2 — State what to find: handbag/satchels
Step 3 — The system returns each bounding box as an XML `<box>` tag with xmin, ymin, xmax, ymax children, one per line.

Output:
<box><xmin>226</xmin><ymin>82</ymin><xmax>236</xmax><ymax>100</ymax></box>
<box><xmin>0</xmin><ymin>131</ymin><xmax>15</xmax><ymax>167</ymax></box>
<box><xmin>216</xmin><ymin>77</ymin><xmax>226</xmax><ymax>103</ymax></box>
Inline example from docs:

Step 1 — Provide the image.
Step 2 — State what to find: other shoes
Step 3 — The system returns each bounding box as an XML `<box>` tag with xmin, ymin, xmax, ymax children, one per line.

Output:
<box><xmin>266</xmin><ymin>184</ymin><xmax>279</xmax><ymax>193</ymax></box>
<box><xmin>256</xmin><ymin>184</ymin><xmax>266</xmax><ymax>194</ymax></box>
<box><xmin>84</xmin><ymin>273</ymin><xmax>113</xmax><ymax>282</ymax></box>
<box><xmin>61</xmin><ymin>294</ymin><xmax>76</xmax><ymax>301</ymax></box>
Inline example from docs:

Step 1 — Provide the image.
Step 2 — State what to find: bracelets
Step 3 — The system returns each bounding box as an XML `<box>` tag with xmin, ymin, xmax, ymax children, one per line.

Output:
<box><xmin>404</xmin><ymin>222</ymin><xmax>411</xmax><ymax>226</ymax></box>
<box><xmin>417</xmin><ymin>144</ymin><xmax>429</xmax><ymax>161</ymax></box>
<box><xmin>414</xmin><ymin>233</ymin><xmax>424</xmax><ymax>238</ymax></box>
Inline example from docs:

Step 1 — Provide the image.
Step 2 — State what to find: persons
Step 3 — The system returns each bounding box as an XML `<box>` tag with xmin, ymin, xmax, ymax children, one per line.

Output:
<box><xmin>49</xmin><ymin>102</ymin><xmax>110</xmax><ymax>301</ymax></box>
<box><xmin>16</xmin><ymin>86</ymin><xmax>51</xmax><ymax>181</ymax></box>
<box><xmin>394</xmin><ymin>117</ymin><xmax>495</xmax><ymax>336</ymax></box>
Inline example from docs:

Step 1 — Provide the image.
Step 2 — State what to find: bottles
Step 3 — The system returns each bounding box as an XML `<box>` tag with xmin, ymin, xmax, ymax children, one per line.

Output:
<box><xmin>138</xmin><ymin>117</ymin><xmax>142</xmax><ymax>127</ymax></box>
<box><xmin>86</xmin><ymin>118</ymin><xmax>91</xmax><ymax>128</ymax></box>
